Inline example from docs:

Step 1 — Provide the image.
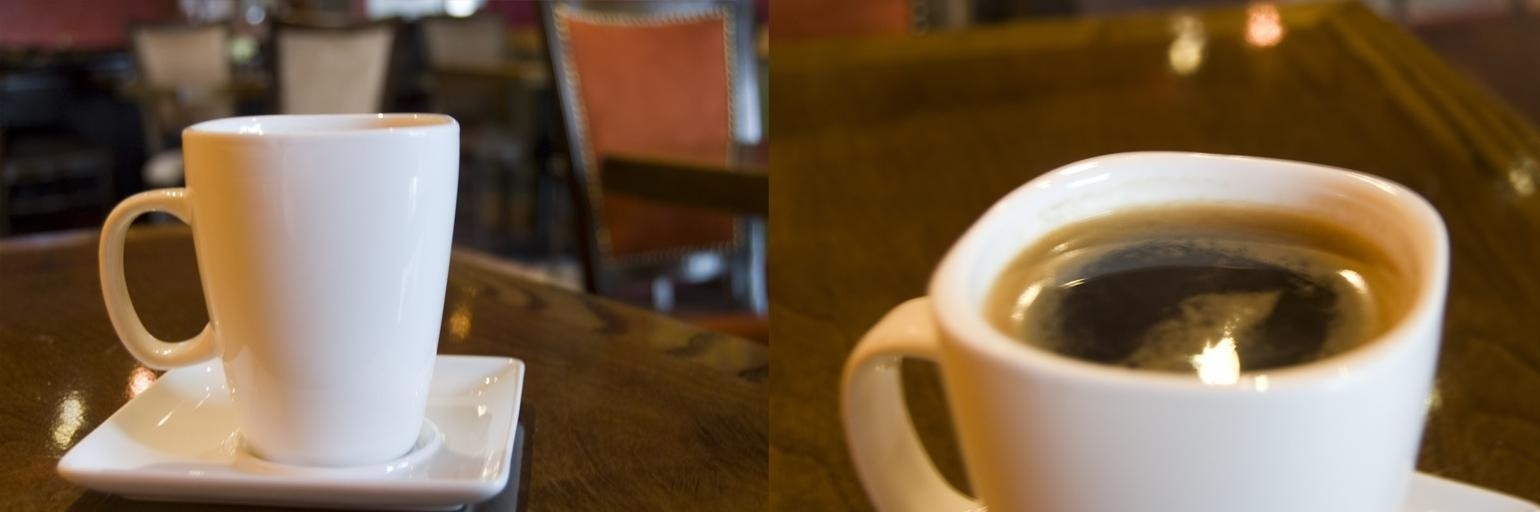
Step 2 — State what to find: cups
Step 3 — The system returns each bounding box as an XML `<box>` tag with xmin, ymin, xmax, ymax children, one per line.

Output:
<box><xmin>840</xmin><ymin>151</ymin><xmax>1450</xmax><ymax>511</ymax></box>
<box><xmin>96</xmin><ymin>114</ymin><xmax>459</xmax><ymax>468</ymax></box>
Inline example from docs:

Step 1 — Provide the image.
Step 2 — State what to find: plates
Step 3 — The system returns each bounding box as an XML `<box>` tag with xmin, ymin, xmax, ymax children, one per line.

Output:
<box><xmin>57</xmin><ymin>354</ymin><xmax>527</xmax><ymax>504</ymax></box>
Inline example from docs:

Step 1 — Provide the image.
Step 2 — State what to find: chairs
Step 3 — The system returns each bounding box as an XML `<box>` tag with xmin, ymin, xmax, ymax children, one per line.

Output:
<box><xmin>115</xmin><ymin>7</ymin><xmax>766</xmax><ymax>350</ymax></box>
